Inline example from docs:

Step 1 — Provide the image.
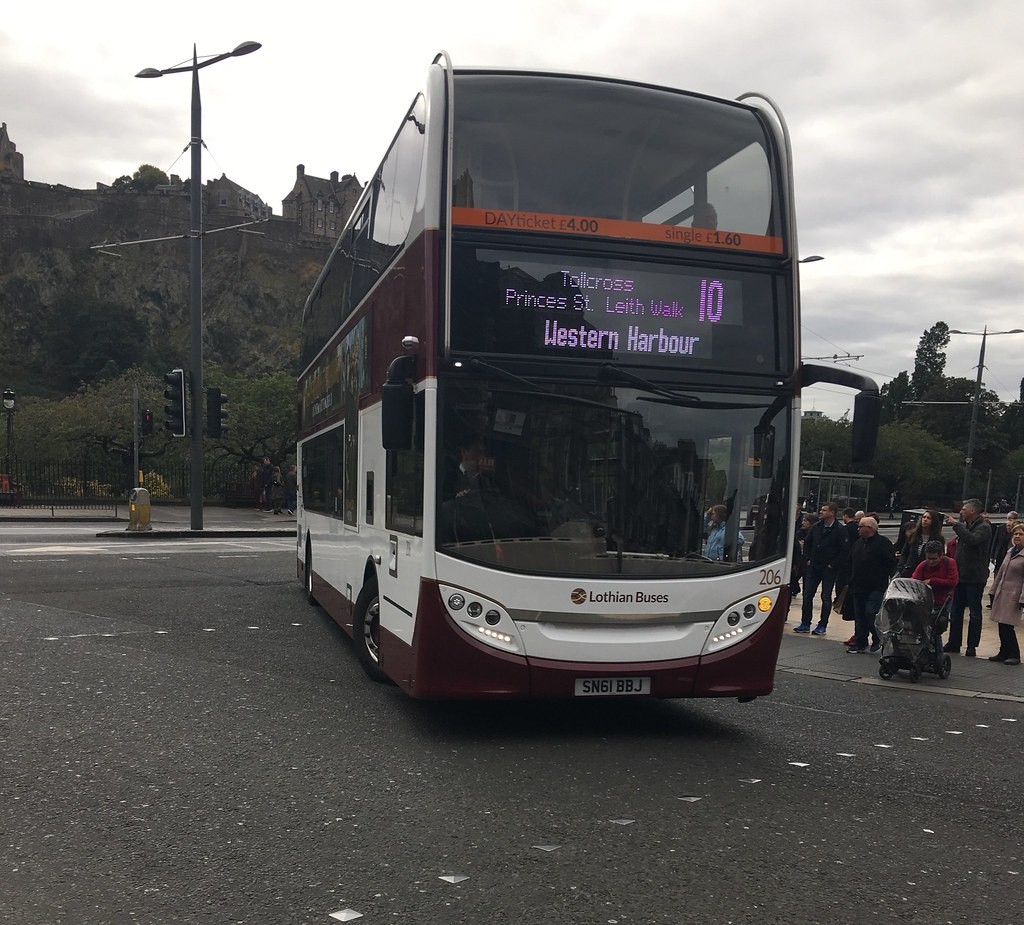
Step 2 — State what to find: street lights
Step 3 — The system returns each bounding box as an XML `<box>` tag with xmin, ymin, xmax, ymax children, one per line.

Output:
<box><xmin>946</xmin><ymin>328</ymin><xmax>1024</xmax><ymax>502</ymax></box>
<box><xmin>132</xmin><ymin>40</ymin><xmax>264</xmax><ymax>529</ymax></box>
<box><xmin>3</xmin><ymin>388</ymin><xmax>17</xmax><ymax>474</ymax></box>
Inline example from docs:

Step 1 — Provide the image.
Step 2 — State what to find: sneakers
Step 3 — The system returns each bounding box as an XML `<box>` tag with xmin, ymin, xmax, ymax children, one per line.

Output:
<box><xmin>844</xmin><ymin>636</ymin><xmax>855</xmax><ymax>646</ymax></box>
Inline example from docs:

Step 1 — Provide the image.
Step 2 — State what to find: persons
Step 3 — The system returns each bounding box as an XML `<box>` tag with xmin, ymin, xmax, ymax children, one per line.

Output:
<box><xmin>833</xmin><ymin>508</ymin><xmax>893</xmax><ymax>654</ymax></box>
<box><xmin>262</xmin><ymin>457</ymin><xmax>274</xmax><ymax>512</ymax></box>
<box><xmin>986</xmin><ymin>510</ymin><xmax>1024</xmax><ymax>664</ymax></box>
<box><xmin>701</xmin><ymin>504</ymin><xmax>744</xmax><ymax>563</ymax></box>
<box><xmin>272</xmin><ymin>466</ymin><xmax>284</xmax><ymax>515</ymax></box>
<box><xmin>286</xmin><ymin>464</ymin><xmax>297</xmax><ymax>515</ymax></box>
<box><xmin>440</xmin><ymin>432</ymin><xmax>499</xmax><ymax>534</ymax></box>
<box><xmin>791</xmin><ymin>502</ymin><xmax>849</xmax><ymax>635</ymax></box>
<box><xmin>893</xmin><ymin>510</ymin><xmax>944</xmax><ymax>579</ymax></box>
<box><xmin>886</xmin><ymin>491</ymin><xmax>899</xmax><ymax>520</ymax></box>
<box><xmin>911</xmin><ymin>540</ymin><xmax>958</xmax><ymax>654</ymax></box>
<box><xmin>806</xmin><ymin>489</ymin><xmax>816</xmax><ymax>513</ymax></box>
<box><xmin>938</xmin><ymin>498</ymin><xmax>993</xmax><ymax>657</ymax></box>
<box><xmin>251</xmin><ymin>463</ymin><xmax>262</xmax><ymax>511</ymax></box>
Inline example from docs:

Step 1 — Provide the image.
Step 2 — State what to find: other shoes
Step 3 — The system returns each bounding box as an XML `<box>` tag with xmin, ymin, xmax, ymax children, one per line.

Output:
<box><xmin>870</xmin><ymin>644</ymin><xmax>882</xmax><ymax>654</ymax></box>
<box><xmin>966</xmin><ymin>647</ymin><xmax>976</xmax><ymax>656</ymax></box>
<box><xmin>943</xmin><ymin>642</ymin><xmax>960</xmax><ymax>653</ymax></box>
<box><xmin>1004</xmin><ymin>657</ymin><xmax>1020</xmax><ymax>664</ymax></box>
<box><xmin>274</xmin><ymin>509</ymin><xmax>281</xmax><ymax>514</ymax></box>
<box><xmin>989</xmin><ymin>653</ymin><xmax>1007</xmax><ymax>661</ymax></box>
<box><xmin>812</xmin><ymin>624</ymin><xmax>826</xmax><ymax>635</ymax></box>
<box><xmin>263</xmin><ymin>508</ymin><xmax>272</xmax><ymax>511</ymax></box>
<box><xmin>793</xmin><ymin>624</ymin><xmax>810</xmax><ymax>633</ymax></box>
<box><xmin>287</xmin><ymin>509</ymin><xmax>294</xmax><ymax>516</ymax></box>
<box><xmin>847</xmin><ymin>646</ymin><xmax>870</xmax><ymax>653</ymax></box>
<box><xmin>255</xmin><ymin>506</ymin><xmax>262</xmax><ymax>510</ymax></box>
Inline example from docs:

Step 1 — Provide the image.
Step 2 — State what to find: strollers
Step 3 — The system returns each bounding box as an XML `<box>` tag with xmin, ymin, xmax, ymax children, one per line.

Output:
<box><xmin>879</xmin><ymin>575</ymin><xmax>954</xmax><ymax>680</ymax></box>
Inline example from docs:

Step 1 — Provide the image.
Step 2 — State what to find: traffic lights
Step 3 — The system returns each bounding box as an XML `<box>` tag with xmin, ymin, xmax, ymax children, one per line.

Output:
<box><xmin>207</xmin><ymin>387</ymin><xmax>230</xmax><ymax>439</ymax></box>
<box><xmin>165</xmin><ymin>370</ymin><xmax>186</xmax><ymax>439</ymax></box>
<box><xmin>142</xmin><ymin>410</ymin><xmax>151</xmax><ymax>434</ymax></box>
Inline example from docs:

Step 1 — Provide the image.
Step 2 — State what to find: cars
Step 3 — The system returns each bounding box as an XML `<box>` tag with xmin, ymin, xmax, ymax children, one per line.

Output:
<box><xmin>746</xmin><ymin>495</ymin><xmax>767</xmax><ymax>525</ymax></box>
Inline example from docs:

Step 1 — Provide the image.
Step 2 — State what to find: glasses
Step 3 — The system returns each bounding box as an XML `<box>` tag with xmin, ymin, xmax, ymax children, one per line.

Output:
<box><xmin>857</xmin><ymin>526</ymin><xmax>870</xmax><ymax>529</ymax></box>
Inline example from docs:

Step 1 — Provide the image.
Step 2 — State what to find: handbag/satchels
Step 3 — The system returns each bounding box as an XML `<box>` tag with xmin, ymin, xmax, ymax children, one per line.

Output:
<box><xmin>833</xmin><ymin>583</ymin><xmax>855</xmax><ymax>620</ymax></box>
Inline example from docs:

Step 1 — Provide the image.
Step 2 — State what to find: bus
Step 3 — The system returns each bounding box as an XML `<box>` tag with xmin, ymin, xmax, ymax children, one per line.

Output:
<box><xmin>295</xmin><ymin>47</ymin><xmax>883</xmax><ymax>706</ymax></box>
